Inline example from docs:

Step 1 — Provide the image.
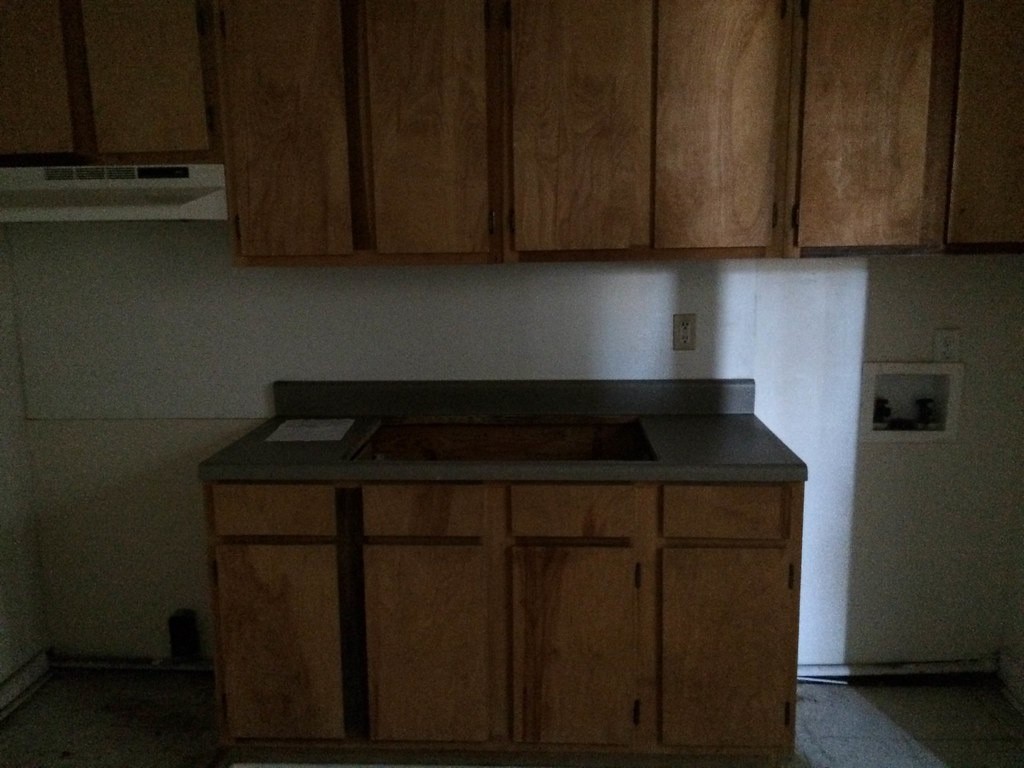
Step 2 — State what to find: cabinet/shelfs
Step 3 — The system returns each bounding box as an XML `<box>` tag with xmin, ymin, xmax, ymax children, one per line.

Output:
<box><xmin>0</xmin><ymin>0</ymin><xmax>1024</xmax><ymax>259</ymax></box>
<box><xmin>202</xmin><ymin>481</ymin><xmax>804</xmax><ymax>763</ymax></box>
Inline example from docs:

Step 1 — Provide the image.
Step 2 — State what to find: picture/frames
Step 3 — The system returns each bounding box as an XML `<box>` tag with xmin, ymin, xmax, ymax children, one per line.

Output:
<box><xmin>861</xmin><ymin>361</ymin><xmax>966</xmax><ymax>444</ymax></box>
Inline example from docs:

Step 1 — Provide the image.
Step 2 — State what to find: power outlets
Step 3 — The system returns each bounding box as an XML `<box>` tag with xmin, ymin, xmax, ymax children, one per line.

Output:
<box><xmin>932</xmin><ymin>327</ymin><xmax>971</xmax><ymax>362</ymax></box>
<box><xmin>672</xmin><ymin>313</ymin><xmax>696</xmax><ymax>351</ymax></box>
